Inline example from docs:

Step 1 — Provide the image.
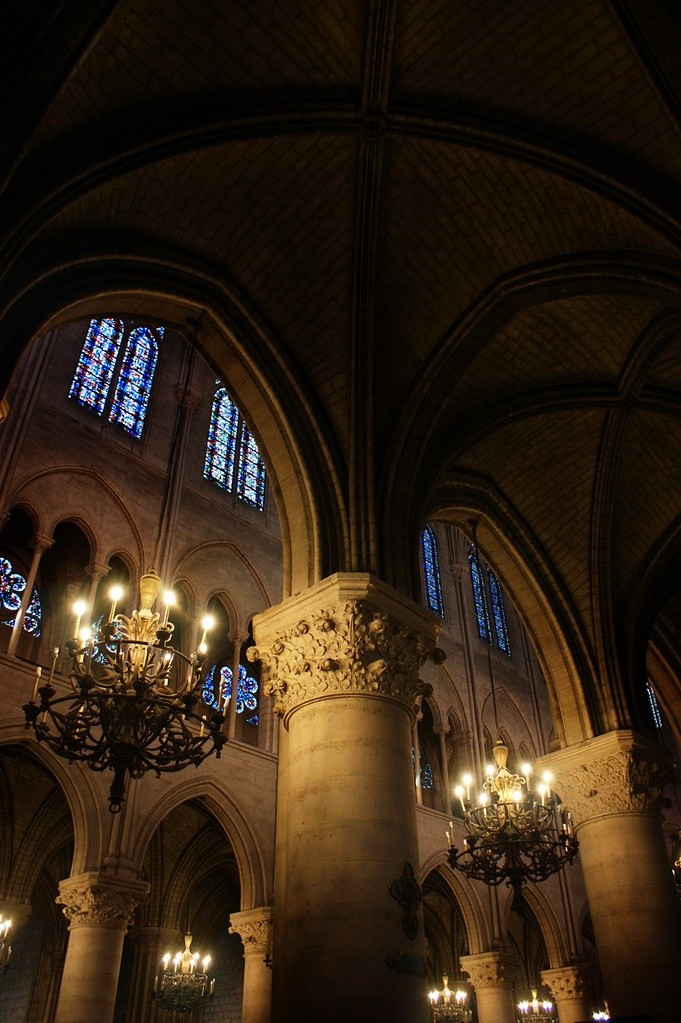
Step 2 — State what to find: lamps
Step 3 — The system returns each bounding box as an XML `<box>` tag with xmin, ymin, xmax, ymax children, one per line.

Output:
<box><xmin>518</xmin><ymin>989</ymin><xmax>553</xmax><ymax>1023</ymax></box>
<box><xmin>21</xmin><ymin>568</ymin><xmax>230</xmax><ymax>814</ymax></box>
<box><xmin>444</xmin><ymin>741</ymin><xmax>580</xmax><ymax>890</ymax></box>
<box><xmin>150</xmin><ymin>930</ymin><xmax>215</xmax><ymax>1011</ymax></box>
<box><xmin>427</xmin><ymin>973</ymin><xmax>473</xmax><ymax>1023</ymax></box>
